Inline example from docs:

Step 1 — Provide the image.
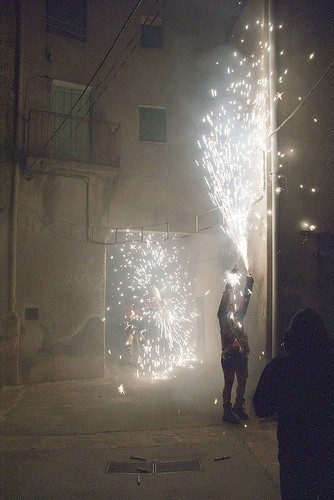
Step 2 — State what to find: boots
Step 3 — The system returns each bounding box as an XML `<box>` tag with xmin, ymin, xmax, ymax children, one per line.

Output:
<box><xmin>232</xmin><ymin>397</ymin><xmax>248</xmax><ymax>420</ymax></box>
<box><xmin>223</xmin><ymin>403</ymin><xmax>239</xmax><ymax>424</ymax></box>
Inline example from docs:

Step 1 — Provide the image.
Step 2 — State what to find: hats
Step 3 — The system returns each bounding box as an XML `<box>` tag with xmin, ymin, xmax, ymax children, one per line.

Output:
<box><xmin>283</xmin><ymin>308</ymin><xmax>330</xmax><ymax>347</ymax></box>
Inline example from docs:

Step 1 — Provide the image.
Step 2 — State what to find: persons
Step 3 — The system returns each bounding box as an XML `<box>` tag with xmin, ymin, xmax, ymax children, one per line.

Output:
<box><xmin>217</xmin><ymin>266</ymin><xmax>255</xmax><ymax>424</ymax></box>
<box><xmin>252</xmin><ymin>307</ymin><xmax>333</xmax><ymax>500</ymax></box>
